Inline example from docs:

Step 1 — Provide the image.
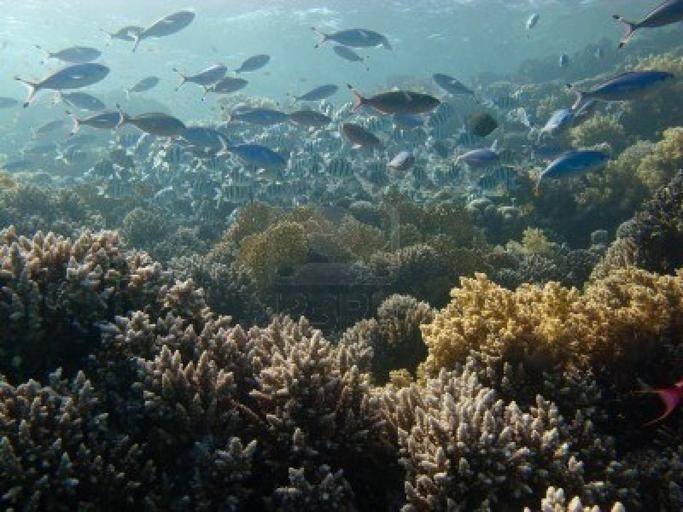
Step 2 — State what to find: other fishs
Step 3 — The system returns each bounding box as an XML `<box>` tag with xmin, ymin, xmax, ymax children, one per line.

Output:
<box><xmin>0</xmin><ymin>9</ymin><xmax>608</xmax><ymax>206</ymax></box>
